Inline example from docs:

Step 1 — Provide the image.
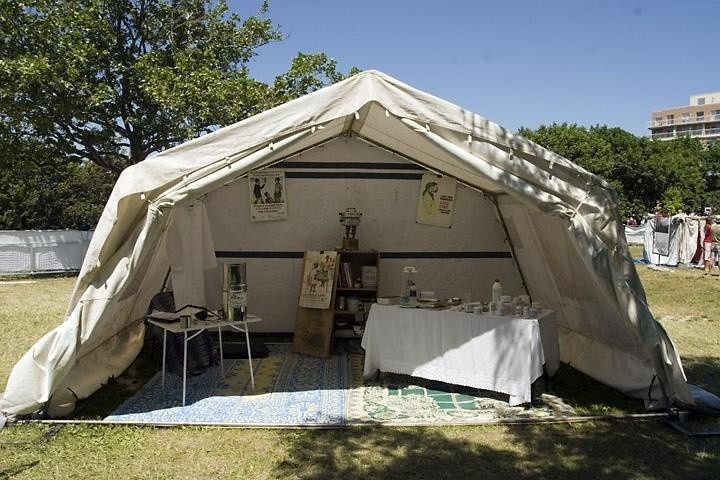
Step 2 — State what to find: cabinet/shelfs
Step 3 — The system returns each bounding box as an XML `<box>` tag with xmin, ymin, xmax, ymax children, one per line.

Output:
<box><xmin>334</xmin><ymin>246</ymin><xmax>382</xmax><ymax>356</ymax></box>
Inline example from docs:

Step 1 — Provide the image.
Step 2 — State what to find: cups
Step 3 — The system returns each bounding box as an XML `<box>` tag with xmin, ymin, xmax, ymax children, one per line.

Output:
<box><xmin>179</xmin><ymin>315</ymin><xmax>191</xmax><ymax>329</ymax></box>
<box><xmin>347</xmin><ymin>298</ymin><xmax>363</xmax><ymax>312</ymax></box>
<box><xmin>473</xmin><ymin>306</ymin><xmax>481</xmax><ymax>315</ymax></box>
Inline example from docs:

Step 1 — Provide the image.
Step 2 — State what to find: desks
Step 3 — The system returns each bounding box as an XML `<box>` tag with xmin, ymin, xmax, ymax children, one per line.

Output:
<box><xmin>370</xmin><ymin>291</ymin><xmax>555</xmax><ymax>412</ymax></box>
<box><xmin>149</xmin><ymin>309</ymin><xmax>265</xmax><ymax>407</ymax></box>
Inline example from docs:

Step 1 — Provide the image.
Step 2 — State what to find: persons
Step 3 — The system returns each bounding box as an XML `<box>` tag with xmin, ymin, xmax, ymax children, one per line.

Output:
<box><xmin>703</xmin><ymin>217</ymin><xmax>714</xmax><ymax>273</ymax></box>
<box><xmin>273</xmin><ymin>177</ymin><xmax>284</xmax><ymax>203</ymax></box>
<box><xmin>253</xmin><ymin>177</ymin><xmax>267</xmax><ymax>204</ymax></box>
<box><xmin>421</xmin><ymin>181</ymin><xmax>439</xmax><ymax>224</ymax></box>
<box><xmin>264</xmin><ymin>191</ymin><xmax>274</xmax><ymax>204</ymax></box>
<box><xmin>706</xmin><ymin>215</ymin><xmax>720</xmax><ymax>276</ymax></box>
<box><xmin>308</xmin><ymin>255</ymin><xmax>335</xmax><ymax>293</ymax></box>
<box><xmin>626</xmin><ymin>216</ymin><xmax>637</xmax><ymax>225</ymax></box>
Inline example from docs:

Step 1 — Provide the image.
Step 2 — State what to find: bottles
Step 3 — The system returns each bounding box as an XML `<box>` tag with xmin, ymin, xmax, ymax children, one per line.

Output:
<box><xmin>409</xmin><ymin>283</ymin><xmax>417</xmax><ymax>306</ymax></box>
<box><xmin>336</xmin><ymin>296</ymin><xmax>345</xmax><ymax>313</ymax></box>
<box><xmin>492</xmin><ymin>279</ymin><xmax>503</xmax><ymax>304</ymax></box>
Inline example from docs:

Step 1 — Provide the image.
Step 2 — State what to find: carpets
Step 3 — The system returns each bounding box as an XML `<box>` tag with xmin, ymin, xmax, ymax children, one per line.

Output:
<box><xmin>104</xmin><ymin>341</ymin><xmax>580</xmax><ymax>426</ymax></box>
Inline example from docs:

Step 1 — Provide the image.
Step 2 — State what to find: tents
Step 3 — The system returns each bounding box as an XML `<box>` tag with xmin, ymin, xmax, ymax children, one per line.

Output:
<box><xmin>2</xmin><ymin>70</ymin><xmax>720</xmax><ymax>417</ymax></box>
